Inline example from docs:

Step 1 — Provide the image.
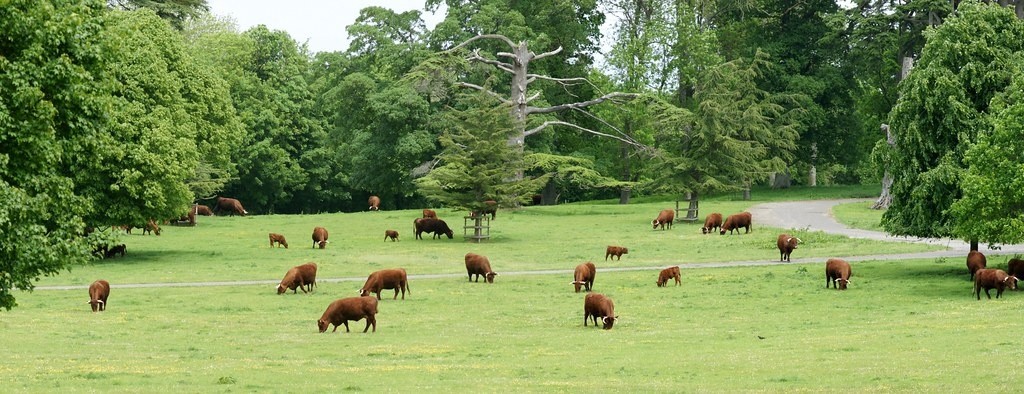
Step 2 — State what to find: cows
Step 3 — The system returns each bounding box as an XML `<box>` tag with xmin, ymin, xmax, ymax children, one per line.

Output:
<box><xmin>93</xmin><ymin>242</ymin><xmax>128</xmax><ymax>257</ymax></box>
<box><xmin>277</xmin><ymin>262</ymin><xmax>317</xmax><ymax>295</ymax></box>
<box><xmin>826</xmin><ymin>259</ymin><xmax>852</xmax><ymax>290</ymax></box>
<box><xmin>317</xmin><ymin>296</ymin><xmax>378</xmax><ymax>333</ymax></box>
<box><xmin>312</xmin><ymin>227</ymin><xmax>329</xmax><ymax>249</ymax></box>
<box><xmin>355</xmin><ymin>269</ymin><xmax>412</xmax><ymax>301</ymax></box>
<box><xmin>368</xmin><ymin>196</ymin><xmax>380</xmax><ymax>211</ymax></box>
<box><xmin>651</xmin><ymin>209</ymin><xmax>752</xmax><ymax>235</ymax></box>
<box><xmin>384</xmin><ymin>230</ymin><xmax>400</xmax><ymax>242</ymax></box>
<box><xmin>465</xmin><ymin>253</ymin><xmax>500</xmax><ymax>283</ymax></box>
<box><xmin>967</xmin><ymin>251</ymin><xmax>1024</xmax><ymax>300</ymax></box>
<box><xmin>86</xmin><ymin>280</ymin><xmax>110</xmax><ymax>312</ymax></box>
<box><xmin>470</xmin><ymin>201</ymin><xmax>498</xmax><ymax>221</ymax></box>
<box><xmin>777</xmin><ymin>234</ymin><xmax>801</xmax><ymax>262</ymax></box>
<box><xmin>413</xmin><ymin>210</ymin><xmax>453</xmax><ymax>240</ymax></box>
<box><xmin>269</xmin><ymin>233</ymin><xmax>288</xmax><ymax>248</ymax></box>
<box><xmin>569</xmin><ymin>246</ymin><xmax>681</xmax><ymax>330</ymax></box>
<box><xmin>214</xmin><ymin>197</ymin><xmax>248</xmax><ymax>217</ymax></box>
<box><xmin>126</xmin><ymin>217</ymin><xmax>161</xmax><ymax>236</ymax></box>
<box><xmin>163</xmin><ymin>204</ymin><xmax>213</xmax><ymax>226</ymax></box>
